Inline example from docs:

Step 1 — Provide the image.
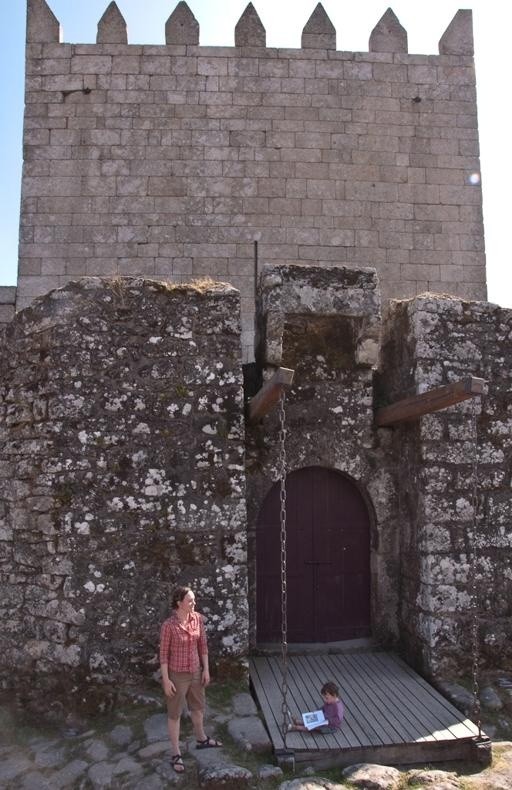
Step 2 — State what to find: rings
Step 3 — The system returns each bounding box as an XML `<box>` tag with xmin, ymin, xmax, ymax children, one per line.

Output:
<box><xmin>207</xmin><ymin>680</ymin><xmax>209</xmax><ymax>682</ymax></box>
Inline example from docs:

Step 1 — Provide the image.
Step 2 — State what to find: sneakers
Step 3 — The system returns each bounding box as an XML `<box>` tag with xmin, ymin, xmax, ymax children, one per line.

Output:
<box><xmin>288</xmin><ymin>724</ymin><xmax>296</xmax><ymax>731</ymax></box>
<box><xmin>288</xmin><ymin>710</ymin><xmax>298</xmax><ymax>725</ymax></box>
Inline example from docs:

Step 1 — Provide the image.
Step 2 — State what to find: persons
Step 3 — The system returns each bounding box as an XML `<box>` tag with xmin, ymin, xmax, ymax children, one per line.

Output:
<box><xmin>286</xmin><ymin>682</ymin><xmax>345</xmax><ymax>735</ymax></box>
<box><xmin>159</xmin><ymin>586</ymin><xmax>224</xmax><ymax>773</ymax></box>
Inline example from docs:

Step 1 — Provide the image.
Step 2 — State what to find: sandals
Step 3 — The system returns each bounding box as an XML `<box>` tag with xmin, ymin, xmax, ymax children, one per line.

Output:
<box><xmin>172</xmin><ymin>754</ymin><xmax>185</xmax><ymax>772</ymax></box>
<box><xmin>197</xmin><ymin>735</ymin><xmax>224</xmax><ymax>748</ymax></box>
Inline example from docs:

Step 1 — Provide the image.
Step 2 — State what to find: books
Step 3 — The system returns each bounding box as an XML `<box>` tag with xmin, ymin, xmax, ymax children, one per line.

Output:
<box><xmin>301</xmin><ymin>709</ymin><xmax>329</xmax><ymax>731</ymax></box>
